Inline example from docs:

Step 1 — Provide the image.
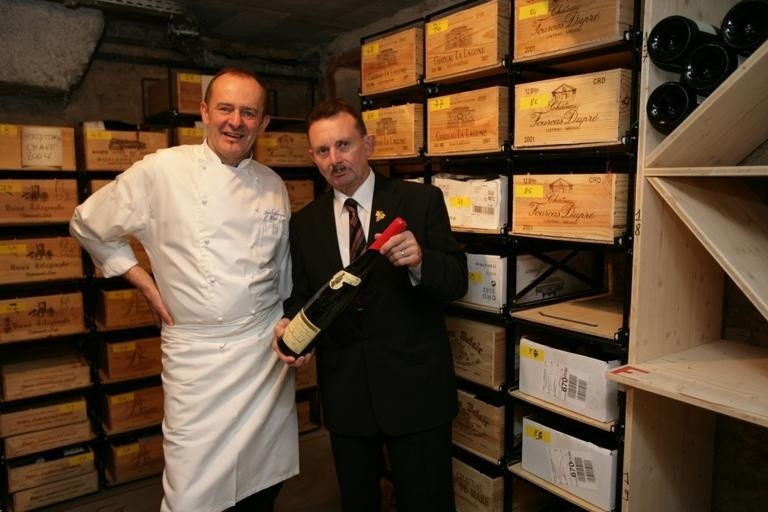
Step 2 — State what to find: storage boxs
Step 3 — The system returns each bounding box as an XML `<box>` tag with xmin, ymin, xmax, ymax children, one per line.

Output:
<box><xmin>0</xmin><ymin>123</ymin><xmax>104</xmax><ymax>512</ymax></box>
<box><xmin>176</xmin><ymin>119</ymin><xmax>320</xmax><ymax>432</ymax></box>
<box><xmin>83</xmin><ymin>121</ymin><xmax>174</xmax><ymax>486</ymax></box>
<box><xmin>145</xmin><ymin>71</ymin><xmax>213</xmax><ymax>120</ymax></box>
<box><xmin>509</xmin><ymin>0</ymin><xmax>633</xmax><ymax>512</ymax></box>
<box><xmin>421</xmin><ymin>0</ymin><xmax>511</xmax><ymax>512</ymax></box>
<box><xmin>360</xmin><ymin>103</ymin><xmax>423</xmax><ymax>161</ymax></box>
<box><xmin>361</xmin><ymin>26</ymin><xmax>424</xmax><ymax>98</ymax></box>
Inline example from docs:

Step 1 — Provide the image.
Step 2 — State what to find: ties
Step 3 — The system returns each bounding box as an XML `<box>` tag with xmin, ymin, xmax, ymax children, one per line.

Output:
<box><xmin>344</xmin><ymin>198</ymin><xmax>367</xmax><ymax>264</ymax></box>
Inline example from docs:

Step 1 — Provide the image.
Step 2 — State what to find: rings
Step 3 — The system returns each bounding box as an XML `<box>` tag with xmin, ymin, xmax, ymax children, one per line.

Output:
<box><xmin>401</xmin><ymin>251</ymin><xmax>407</xmax><ymax>257</ymax></box>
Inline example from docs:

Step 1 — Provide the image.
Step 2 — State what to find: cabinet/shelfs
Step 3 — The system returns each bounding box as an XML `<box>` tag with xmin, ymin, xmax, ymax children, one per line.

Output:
<box><xmin>622</xmin><ymin>0</ymin><xmax>768</xmax><ymax>512</ymax></box>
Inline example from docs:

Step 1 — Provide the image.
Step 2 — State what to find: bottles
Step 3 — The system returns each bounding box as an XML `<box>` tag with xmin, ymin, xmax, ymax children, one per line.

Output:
<box><xmin>645</xmin><ymin>82</ymin><xmax>713</xmax><ymax>136</ymax></box>
<box><xmin>79</xmin><ymin>119</ymin><xmax>149</xmax><ymax>132</ymax></box>
<box><xmin>681</xmin><ymin>41</ymin><xmax>747</xmax><ymax>99</ymax></box>
<box><xmin>647</xmin><ymin>15</ymin><xmax>724</xmax><ymax>74</ymax></box>
<box><xmin>277</xmin><ymin>216</ymin><xmax>408</xmax><ymax>361</ymax></box>
<box><xmin>144</xmin><ymin>107</ymin><xmax>180</xmax><ymax>130</ymax></box>
<box><xmin>719</xmin><ymin>1</ymin><xmax>767</xmax><ymax>59</ymax></box>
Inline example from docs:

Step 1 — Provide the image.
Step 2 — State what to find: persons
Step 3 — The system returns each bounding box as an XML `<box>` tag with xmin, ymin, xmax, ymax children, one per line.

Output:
<box><xmin>70</xmin><ymin>70</ymin><xmax>293</xmax><ymax>512</ymax></box>
<box><xmin>273</xmin><ymin>98</ymin><xmax>469</xmax><ymax>509</ymax></box>
